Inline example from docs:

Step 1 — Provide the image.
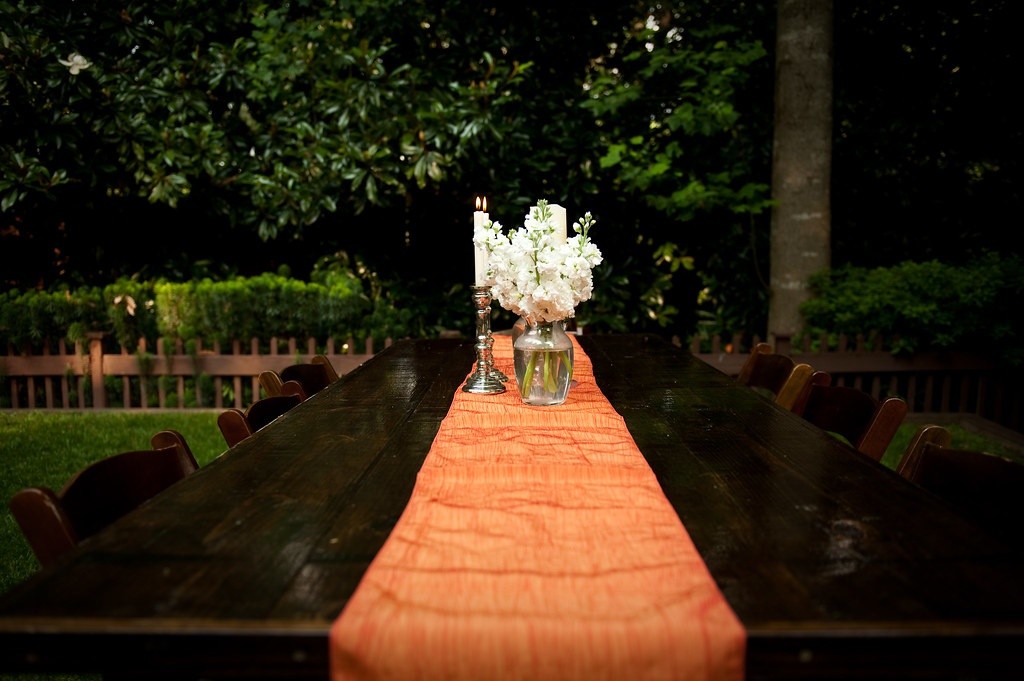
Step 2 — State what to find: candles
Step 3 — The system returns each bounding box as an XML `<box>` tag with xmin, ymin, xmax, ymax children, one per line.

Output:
<box><xmin>529</xmin><ymin>204</ymin><xmax>567</xmax><ymax>243</ymax></box>
<box><xmin>474</xmin><ymin>211</ymin><xmax>489</xmax><ymax>285</ymax></box>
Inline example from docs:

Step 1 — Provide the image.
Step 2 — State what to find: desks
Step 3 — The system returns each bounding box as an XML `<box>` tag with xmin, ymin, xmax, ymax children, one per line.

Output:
<box><xmin>0</xmin><ymin>336</ymin><xmax>1024</xmax><ymax>681</ymax></box>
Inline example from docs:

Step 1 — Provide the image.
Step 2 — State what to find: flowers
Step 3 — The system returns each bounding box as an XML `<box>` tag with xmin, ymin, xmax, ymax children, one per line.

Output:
<box><xmin>473</xmin><ymin>199</ymin><xmax>604</xmax><ymax>396</ymax></box>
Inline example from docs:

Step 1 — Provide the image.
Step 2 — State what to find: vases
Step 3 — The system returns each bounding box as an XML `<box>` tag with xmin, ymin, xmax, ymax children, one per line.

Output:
<box><xmin>514</xmin><ymin>318</ymin><xmax>574</xmax><ymax>406</ymax></box>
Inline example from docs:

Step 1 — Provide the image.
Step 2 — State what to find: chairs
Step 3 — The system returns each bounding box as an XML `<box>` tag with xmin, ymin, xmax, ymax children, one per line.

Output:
<box><xmin>738</xmin><ymin>343</ymin><xmax>1024</xmax><ymax>543</ymax></box>
<box><xmin>11</xmin><ymin>353</ymin><xmax>337</xmax><ymax>565</ymax></box>
